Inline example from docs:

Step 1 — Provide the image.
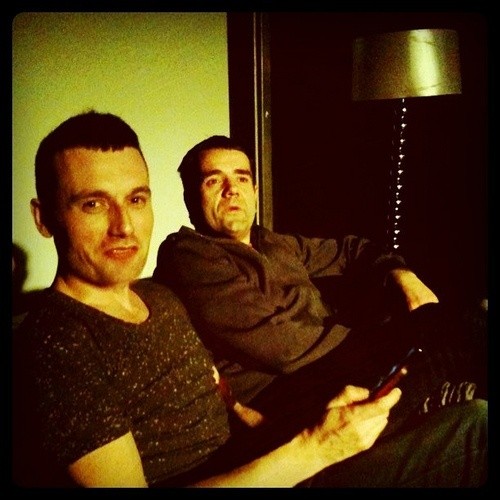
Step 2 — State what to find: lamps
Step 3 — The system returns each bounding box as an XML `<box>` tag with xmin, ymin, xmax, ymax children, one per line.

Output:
<box><xmin>352</xmin><ymin>26</ymin><xmax>463</xmax><ymax>254</ymax></box>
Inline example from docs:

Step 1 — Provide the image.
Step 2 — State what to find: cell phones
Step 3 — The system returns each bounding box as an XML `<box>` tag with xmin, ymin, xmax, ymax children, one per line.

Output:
<box><xmin>350</xmin><ymin>345</ymin><xmax>424</xmax><ymax>406</ymax></box>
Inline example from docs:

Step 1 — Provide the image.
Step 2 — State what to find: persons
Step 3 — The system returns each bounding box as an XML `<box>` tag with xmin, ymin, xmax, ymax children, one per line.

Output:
<box><xmin>0</xmin><ymin>111</ymin><xmax>499</xmax><ymax>498</ymax></box>
<box><xmin>151</xmin><ymin>134</ymin><xmax>442</xmax><ymax>433</ymax></box>
